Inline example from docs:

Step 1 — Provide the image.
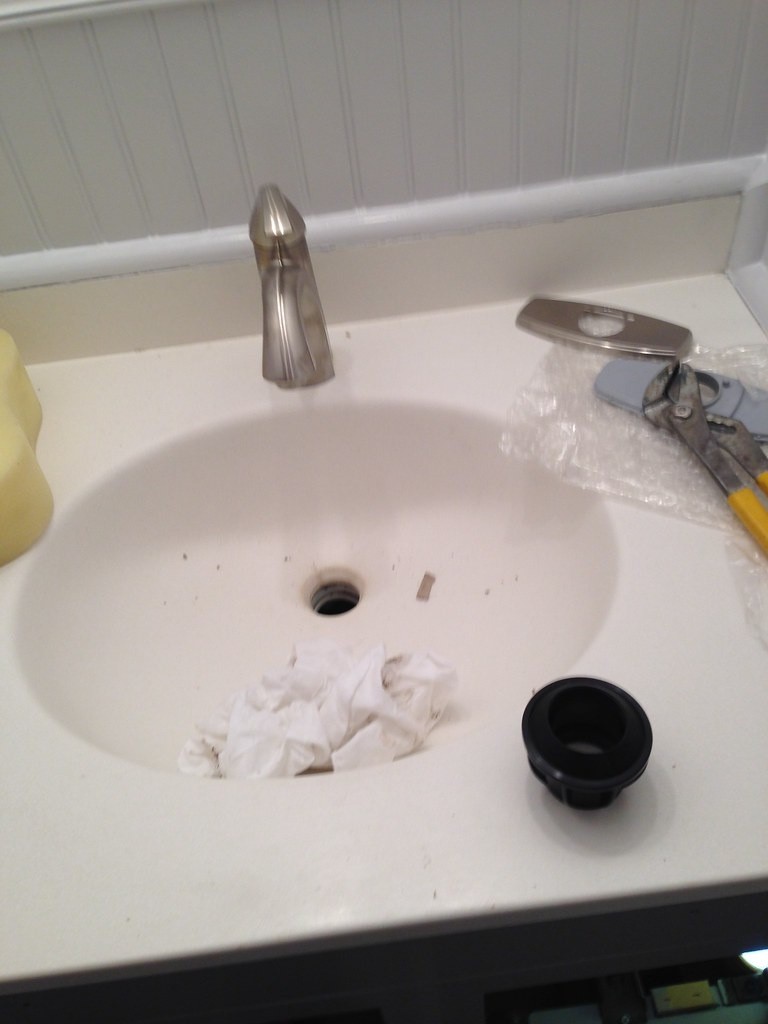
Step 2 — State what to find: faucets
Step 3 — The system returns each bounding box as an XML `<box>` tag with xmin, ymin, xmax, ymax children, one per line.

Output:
<box><xmin>244</xmin><ymin>181</ymin><xmax>337</xmax><ymax>394</ymax></box>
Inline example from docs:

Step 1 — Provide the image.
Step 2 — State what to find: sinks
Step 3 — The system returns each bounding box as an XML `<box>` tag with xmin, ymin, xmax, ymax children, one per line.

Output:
<box><xmin>0</xmin><ymin>274</ymin><xmax>768</xmax><ymax>1006</ymax></box>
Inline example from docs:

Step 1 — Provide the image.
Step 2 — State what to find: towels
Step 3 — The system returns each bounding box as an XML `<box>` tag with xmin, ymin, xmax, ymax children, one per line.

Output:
<box><xmin>174</xmin><ymin>641</ymin><xmax>449</xmax><ymax>786</ymax></box>
